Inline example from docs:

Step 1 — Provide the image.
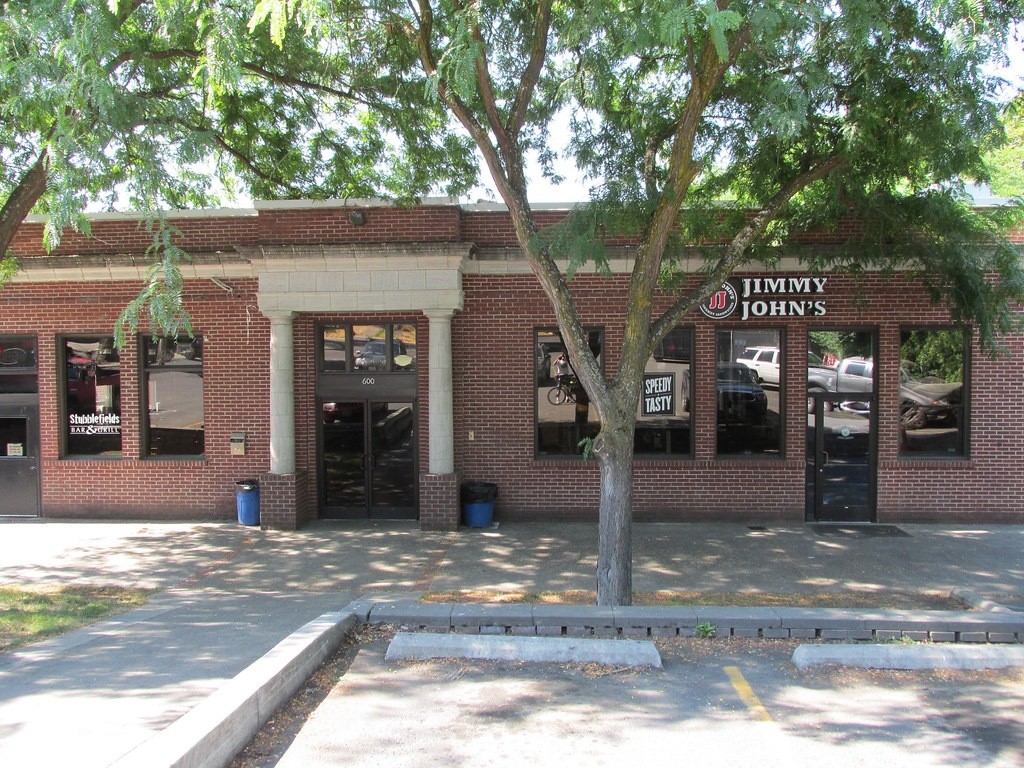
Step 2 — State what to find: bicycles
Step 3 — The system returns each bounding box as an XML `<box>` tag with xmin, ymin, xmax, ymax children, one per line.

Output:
<box><xmin>547</xmin><ymin>372</ymin><xmax>577</xmax><ymax>406</ymax></box>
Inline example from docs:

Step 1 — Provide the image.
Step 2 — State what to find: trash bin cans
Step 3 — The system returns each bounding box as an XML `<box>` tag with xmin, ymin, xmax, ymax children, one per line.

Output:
<box><xmin>234</xmin><ymin>478</ymin><xmax>260</xmax><ymax>527</ymax></box>
<box><xmin>460</xmin><ymin>481</ymin><xmax>499</xmax><ymax>530</ymax></box>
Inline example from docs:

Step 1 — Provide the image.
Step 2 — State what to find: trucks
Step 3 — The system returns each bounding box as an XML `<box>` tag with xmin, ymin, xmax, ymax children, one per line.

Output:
<box><xmin>66</xmin><ymin>356</ymin><xmax>120</xmax><ymax>402</ymax></box>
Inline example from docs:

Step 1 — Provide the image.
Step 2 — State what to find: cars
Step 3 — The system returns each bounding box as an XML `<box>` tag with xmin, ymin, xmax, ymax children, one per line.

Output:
<box><xmin>147</xmin><ymin>335</ymin><xmax>178</xmax><ymax>362</ymax></box>
<box><xmin>323</xmin><ymin>401</ymin><xmax>388</xmax><ymax>424</ymax></box>
<box><xmin>537</xmin><ymin>340</ymin><xmax>551</xmax><ymax>385</ymax></box>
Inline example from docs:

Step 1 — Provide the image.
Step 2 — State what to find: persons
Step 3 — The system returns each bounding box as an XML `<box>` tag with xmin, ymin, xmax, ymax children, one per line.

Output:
<box><xmin>553</xmin><ymin>354</ymin><xmax>572</xmax><ymax>404</ymax></box>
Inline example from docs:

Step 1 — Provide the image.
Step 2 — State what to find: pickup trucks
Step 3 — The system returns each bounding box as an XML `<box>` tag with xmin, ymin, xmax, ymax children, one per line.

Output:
<box><xmin>808</xmin><ymin>355</ymin><xmax>964</xmax><ymax>428</ymax></box>
<box><xmin>680</xmin><ymin>361</ymin><xmax>768</xmax><ymax>422</ymax></box>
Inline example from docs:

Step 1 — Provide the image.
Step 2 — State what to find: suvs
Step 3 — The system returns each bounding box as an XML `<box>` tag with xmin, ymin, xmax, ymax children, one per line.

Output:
<box><xmin>324</xmin><ymin>336</ymin><xmax>362</xmax><ymax>372</ymax></box>
<box><xmin>735</xmin><ymin>344</ymin><xmax>824</xmax><ymax>389</ymax></box>
<box><xmin>352</xmin><ymin>339</ymin><xmax>412</xmax><ymax>372</ymax></box>
